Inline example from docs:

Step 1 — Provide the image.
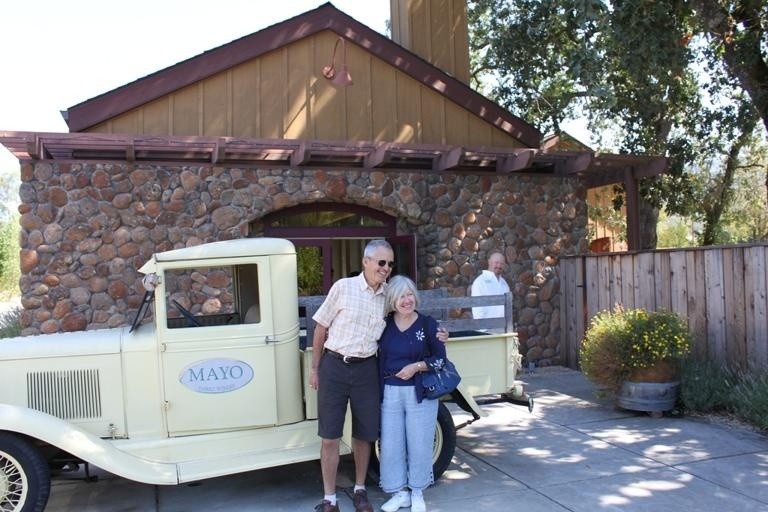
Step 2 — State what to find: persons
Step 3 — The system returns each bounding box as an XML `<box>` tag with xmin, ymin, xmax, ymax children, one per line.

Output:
<box><xmin>471</xmin><ymin>252</ymin><xmax>512</xmax><ymax>335</ymax></box>
<box><xmin>324</xmin><ymin>274</ymin><xmax>448</xmax><ymax>512</ymax></box>
<box><xmin>307</xmin><ymin>240</ymin><xmax>450</xmax><ymax>512</ymax></box>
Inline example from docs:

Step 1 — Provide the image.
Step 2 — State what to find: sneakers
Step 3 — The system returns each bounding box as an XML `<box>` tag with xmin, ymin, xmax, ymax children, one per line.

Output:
<box><xmin>314</xmin><ymin>498</ymin><xmax>341</xmax><ymax>512</ymax></box>
<box><xmin>410</xmin><ymin>489</ymin><xmax>425</xmax><ymax>512</ymax></box>
<box><xmin>381</xmin><ymin>490</ymin><xmax>412</xmax><ymax>512</ymax></box>
<box><xmin>353</xmin><ymin>489</ymin><xmax>373</xmax><ymax>512</ymax></box>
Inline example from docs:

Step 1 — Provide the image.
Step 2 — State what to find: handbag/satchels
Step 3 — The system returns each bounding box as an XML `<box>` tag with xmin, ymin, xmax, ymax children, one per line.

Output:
<box><xmin>421</xmin><ymin>358</ymin><xmax>462</xmax><ymax>400</ymax></box>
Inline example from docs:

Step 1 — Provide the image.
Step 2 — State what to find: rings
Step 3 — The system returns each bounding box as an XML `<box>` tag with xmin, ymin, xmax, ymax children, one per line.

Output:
<box><xmin>403</xmin><ymin>377</ymin><xmax>406</xmax><ymax>379</ymax></box>
<box><xmin>309</xmin><ymin>384</ymin><xmax>312</xmax><ymax>387</ymax></box>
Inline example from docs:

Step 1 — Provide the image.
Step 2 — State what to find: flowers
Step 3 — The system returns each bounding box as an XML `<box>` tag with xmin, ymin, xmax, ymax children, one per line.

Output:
<box><xmin>581</xmin><ymin>301</ymin><xmax>692</xmax><ymax>398</ymax></box>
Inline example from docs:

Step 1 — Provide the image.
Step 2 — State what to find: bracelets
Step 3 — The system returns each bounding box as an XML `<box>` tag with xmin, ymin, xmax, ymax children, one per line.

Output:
<box><xmin>310</xmin><ymin>369</ymin><xmax>319</xmax><ymax>373</ymax></box>
<box><xmin>417</xmin><ymin>362</ymin><xmax>422</xmax><ymax>373</ymax></box>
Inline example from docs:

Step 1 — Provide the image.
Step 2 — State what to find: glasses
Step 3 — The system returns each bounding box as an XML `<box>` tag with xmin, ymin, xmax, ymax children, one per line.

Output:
<box><xmin>369</xmin><ymin>259</ymin><xmax>394</xmax><ymax>269</ymax></box>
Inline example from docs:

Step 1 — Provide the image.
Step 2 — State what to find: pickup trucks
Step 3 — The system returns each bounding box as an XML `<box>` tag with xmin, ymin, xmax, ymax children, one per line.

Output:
<box><xmin>0</xmin><ymin>237</ymin><xmax>534</xmax><ymax>512</ymax></box>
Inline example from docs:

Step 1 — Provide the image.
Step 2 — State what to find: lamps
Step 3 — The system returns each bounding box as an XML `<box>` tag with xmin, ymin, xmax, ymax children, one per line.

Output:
<box><xmin>322</xmin><ymin>38</ymin><xmax>354</xmax><ymax>86</ymax></box>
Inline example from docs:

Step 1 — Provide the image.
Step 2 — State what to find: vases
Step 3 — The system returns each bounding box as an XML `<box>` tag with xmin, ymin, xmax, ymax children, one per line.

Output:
<box><xmin>613</xmin><ymin>357</ymin><xmax>682</xmax><ymax>418</ymax></box>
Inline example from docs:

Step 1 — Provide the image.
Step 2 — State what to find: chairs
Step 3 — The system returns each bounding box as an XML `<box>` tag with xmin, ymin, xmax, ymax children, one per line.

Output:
<box><xmin>245</xmin><ymin>304</ymin><xmax>261</xmax><ymax>323</ymax></box>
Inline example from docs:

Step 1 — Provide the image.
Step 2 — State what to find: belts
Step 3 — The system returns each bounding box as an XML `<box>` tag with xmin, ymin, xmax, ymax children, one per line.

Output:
<box><xmin>323</xmin><ymin>347</ymin><xmax>377</xmax><ymax>364</ymax></box>
